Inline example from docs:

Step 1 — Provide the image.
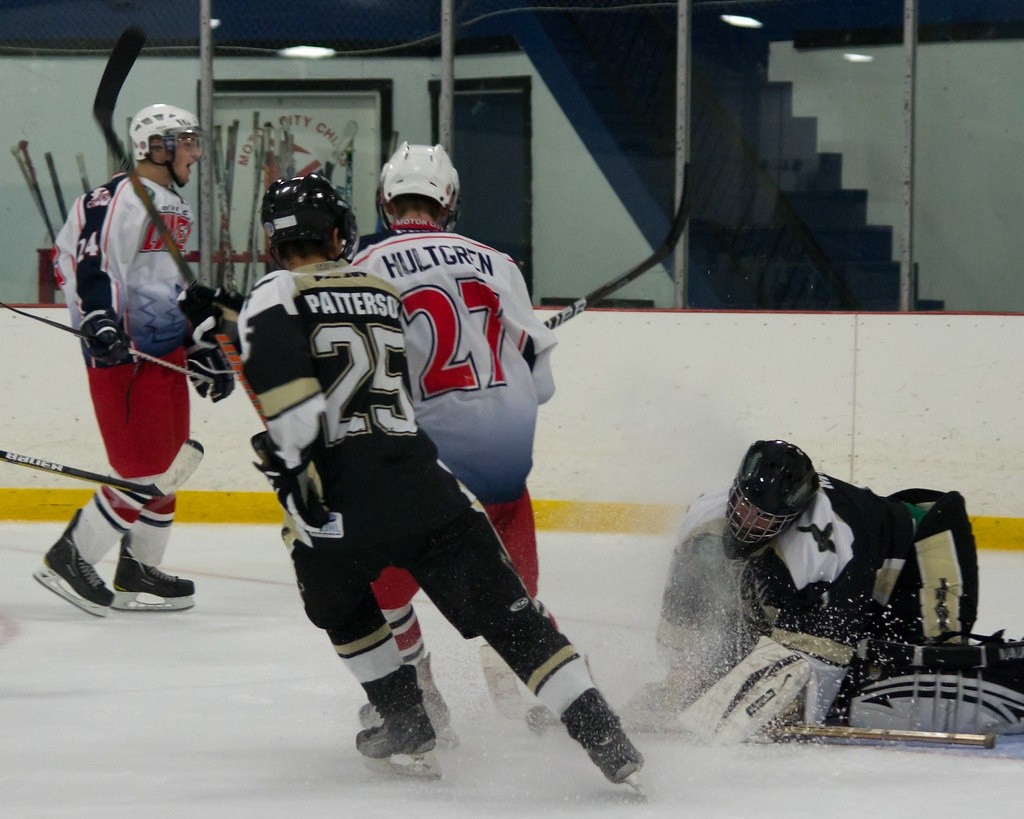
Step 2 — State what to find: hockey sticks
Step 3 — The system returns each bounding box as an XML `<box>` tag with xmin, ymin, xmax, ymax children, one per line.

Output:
<box><xmin>94</xmin><ymin>24</ymin><xmax>271</xmax><ymax>433</ymax></box>
<box><xmin>547</xmin><ymin>157</ymin><xmax>699</xmax><ymax>332</ymax></box>
<box><xmin>0</xmin><ymin>298</ymin><xmax>216</xmax><ymax>382</ymax></box>
<box><xmin>11</xmin><ymin>108</ymin><xmax>357</xmax><ymax>298</ymax></box>
<box><xmin>1</xmin><ymin>437</ymin><xmax>205</xmax><ymax>497</ymax></box>
<box><xmin>478</xmin><ymin>644</ymin><xmax>995</xmax><ymax>750</ymax></box>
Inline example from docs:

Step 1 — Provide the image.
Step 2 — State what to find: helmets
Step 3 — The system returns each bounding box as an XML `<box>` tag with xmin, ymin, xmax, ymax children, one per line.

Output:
<box><xmin>723</xmin><ymin>439</ymin><xmax>819</xmax><ymax>560</ymax></box>
<box><xmin>374</xmin><ymin>142</ymin><xmax>462</xmax><ymax>230</ymax></box>
<box><xmin>260</xmin><ymin>173</ymin><xmax>361</xmax><ymax>264</ymax></box>
<box><xmin>127</xmin><ymin>102</ymin><xmax>199</xmax><ymax>163</ymax></box>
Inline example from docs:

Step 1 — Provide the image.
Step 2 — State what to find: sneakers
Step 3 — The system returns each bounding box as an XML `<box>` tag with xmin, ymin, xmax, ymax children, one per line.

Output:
<box><xmin>357</xmin><ymin>655</ymin><xmax>460</xmax><ymax>749</ymax></box>
<box><xmin>354</xmin><ymin>664</ymin><xmax>444</xmax><ymax>780</ymax></box>
<box><xmin>32</xmin><ymin>506</ymin><xmax>116</xmax><ymax>617</ymax></box>
<box><xmin>479</xmin><ymin>644</ymin><xmax>526</xmax><ymax>716</ymax></box>
<box><xmin>559</xmin><ymin>688</ymin><xmax>648</xmax><ymax>801</ymax></box>
<box><xmin>113</xmin><ymin>535</ymin><xmax>197</xmax><ymax>613</ymax></box>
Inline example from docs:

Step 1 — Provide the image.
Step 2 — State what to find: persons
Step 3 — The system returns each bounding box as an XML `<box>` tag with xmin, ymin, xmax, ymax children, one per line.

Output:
<box><xmin>612</xmin><ymin>435</ymin><xmax>981</xmax><ymax>740</ymax></box>
<box><xmin>342</xmin><ymin>142</ymin><xmax>561</xmax><ymax>742</ymax></box>
<box><xmin>31</xmin><ymin>101</ymin><xmax>235</xmax><ymax>618</ymax></box>
<box><xmin>180</xmin><ymin>170</ymin><xmax>647</xmax><ymax>799</ymax></box>
